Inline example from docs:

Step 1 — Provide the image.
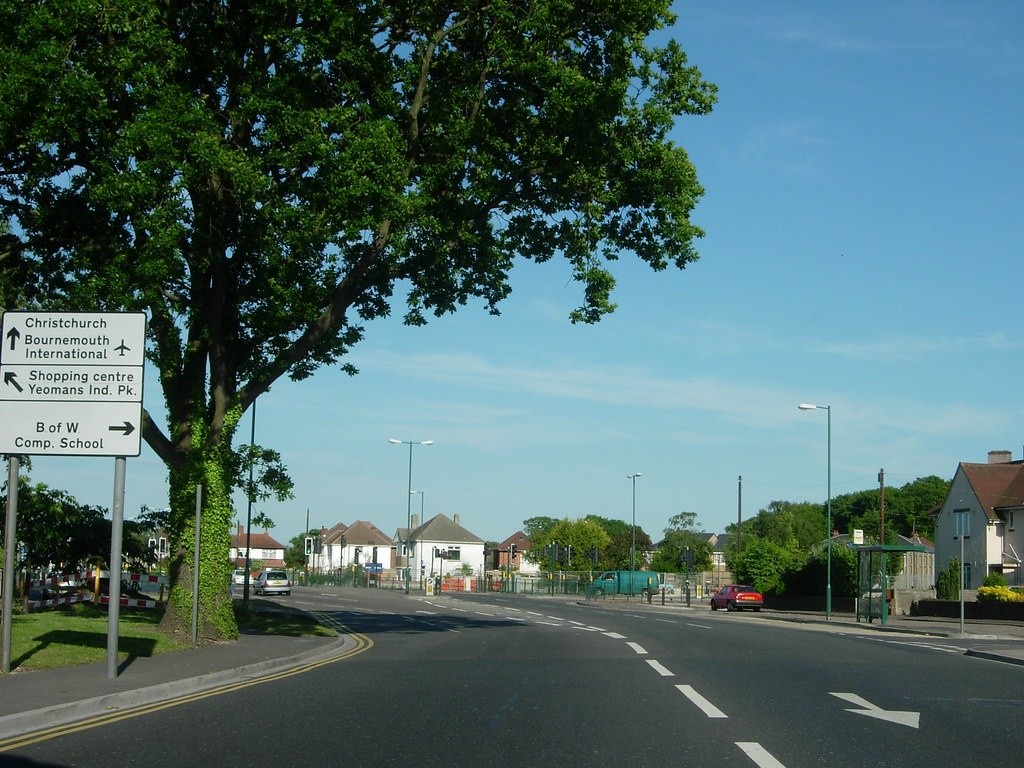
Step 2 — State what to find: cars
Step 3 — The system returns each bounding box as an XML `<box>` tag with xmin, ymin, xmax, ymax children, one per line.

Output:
<box><xmin>230</xmin><ymin>569</ymin><xmax>255</xmax><ymax>585</ymax></box>
<box><xmin>710</xmin><ymin>584</ymin><xmax>764</xmax><ymax>612</ymax></box>
<box><xmin>253</xmin><ymin>570</ymin><xmax>292</xmax><ymax>596</ymax></box>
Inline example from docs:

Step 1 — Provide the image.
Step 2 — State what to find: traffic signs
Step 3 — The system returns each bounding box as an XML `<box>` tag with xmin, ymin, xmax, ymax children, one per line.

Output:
<box><xmin>0</xmin><ymin>310</ymin><xmax>148</xmax><ymax>458</ymax></box>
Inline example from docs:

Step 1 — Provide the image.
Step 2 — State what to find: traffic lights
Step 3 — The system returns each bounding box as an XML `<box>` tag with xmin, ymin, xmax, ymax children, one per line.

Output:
<box><xmin>512</xmin><ymin>545</ymin><xmax>518</xmax><ymax>559</ymax></box>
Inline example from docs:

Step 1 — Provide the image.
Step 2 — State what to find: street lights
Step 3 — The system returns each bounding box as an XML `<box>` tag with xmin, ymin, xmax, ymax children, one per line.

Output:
<box><xmin>304</xmin><ymin>538</ymin><xmax>315</xmax><ymax>556</ymax></box>
<box><xmin>626</xmin><ymin>473</ymin><xmax>644</xmax><ymax>596</ymax></box>
<box><xmin>388</xmin><ymin>439</ymin><xmax>434</xmax><ymax>594</ymax></box>
<box><xmin>797</xmin><ymin>403</ymin><xmax>832</xmax><ymax>621</ymax></box>
<box><xmin>410</xmin><ymin>490</ymin><xmax>424</xmax><ymax>589</ymax></box>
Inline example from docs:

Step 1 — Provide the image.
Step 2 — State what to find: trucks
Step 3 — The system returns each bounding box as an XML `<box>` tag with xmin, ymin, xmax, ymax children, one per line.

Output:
<box><xmin>588</xmin><ymin>570</ymin><xmax>660</xmax><ymax>596</ymax></box>
